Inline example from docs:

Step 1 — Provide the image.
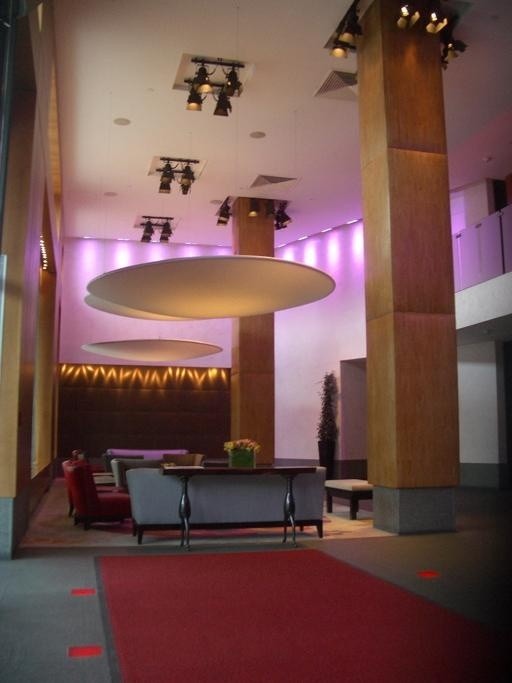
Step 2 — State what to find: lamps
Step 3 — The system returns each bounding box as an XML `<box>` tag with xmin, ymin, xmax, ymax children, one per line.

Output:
<box><xmin>186</xmin><ymin>59</ymin><xmax>242</xmax><ymax>118</ymax></box>
<box><xmin>217</xmin><ymin>198</ymin><xmax>291</xmax><ymax>229</ymax></box>
<box><xmin>141</xmin><ymin>215</ymin><xmax>172</xmax><ymax>245</ymax></box>
<box><xmin>156</xmin><ymin>157</ymin><xmax>198</xmax><ymax>194</ymax></box>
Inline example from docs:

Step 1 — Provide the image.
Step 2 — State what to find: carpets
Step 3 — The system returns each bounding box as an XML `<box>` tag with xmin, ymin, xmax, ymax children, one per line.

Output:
<box><xmin>89</xmin><ymin>544</ymin><xmax>511</xmax><ymax>682</ymax></box>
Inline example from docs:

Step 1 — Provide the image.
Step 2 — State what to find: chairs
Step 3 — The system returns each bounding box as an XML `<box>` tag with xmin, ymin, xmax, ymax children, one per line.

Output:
<box><xmin>62</xmin><ymin>458</ymin><xmax>131</xmax><ymax>532</ymax></box>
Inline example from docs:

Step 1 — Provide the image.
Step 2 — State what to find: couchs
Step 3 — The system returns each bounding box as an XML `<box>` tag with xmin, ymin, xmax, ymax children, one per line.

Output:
<box><xmin>112</xmin><ymin>455</ymin><xmax>327</xmax><ymax>545</ymax></box>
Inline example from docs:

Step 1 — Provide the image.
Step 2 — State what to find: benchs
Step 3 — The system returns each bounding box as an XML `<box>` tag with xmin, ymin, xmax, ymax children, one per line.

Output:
<box><xmin>325</xmin><ymin>479</ymin><xmax>373</xmax><ymax>521</ymax></box>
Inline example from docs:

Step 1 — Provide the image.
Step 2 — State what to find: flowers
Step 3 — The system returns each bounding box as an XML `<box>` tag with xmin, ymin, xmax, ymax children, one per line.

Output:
<box><xmin>222</xmin><ymin>439</ymin><xmax>261</xmax><ymax>454</ymax></box>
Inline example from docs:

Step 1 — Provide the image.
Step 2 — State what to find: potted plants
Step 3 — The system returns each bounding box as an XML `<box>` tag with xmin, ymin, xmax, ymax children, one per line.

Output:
<box><xmin>316</xmin><ymin>370</ymin><xmax>338</xmax><ymax>479</ymax></box>
